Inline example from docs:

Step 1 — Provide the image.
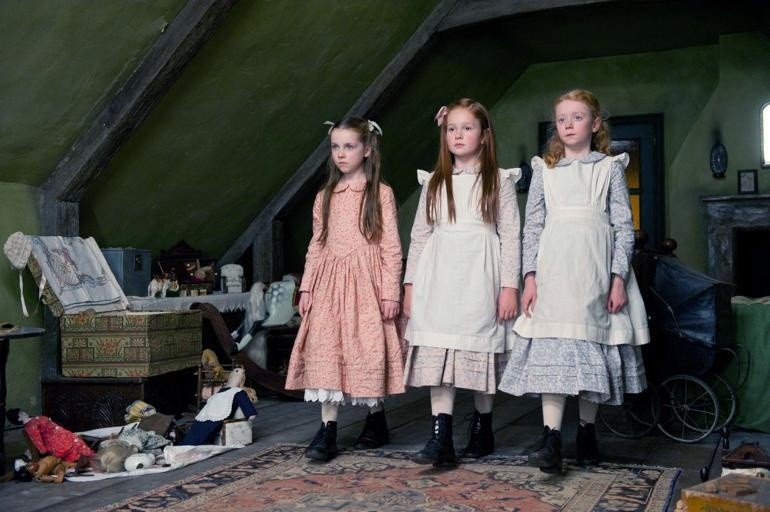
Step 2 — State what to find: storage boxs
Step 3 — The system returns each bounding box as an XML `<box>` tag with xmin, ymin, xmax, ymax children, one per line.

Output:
<box><xmin>672</xmin><ymin>472</ymin><xmax>770</xmax><ymax>511</ymax></box>
<box><xmin>38</xmin><ymin>366</ymin><xmax>200</xmax><ymax>458</ymax></box>
<box><xmin>5</xmin><ymin>232</ymin><xmax>204</xmax><ymax>378</ymax></box>
<box><xmin>98</xmin><ymin>245</ymin><xmax>153</xmax><ymax>298</ymax></box>
<box><xmin>218</xmin><ymin>417</ymin><xmax>254</xmax><ymax>448</ymax></box>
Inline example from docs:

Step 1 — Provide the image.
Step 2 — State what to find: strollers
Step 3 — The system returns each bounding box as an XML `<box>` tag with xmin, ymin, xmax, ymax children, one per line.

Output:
<box><xmin>595</xmin><ymin>235</ymin><xmax>743</xmax><ymax>444</ymax></box>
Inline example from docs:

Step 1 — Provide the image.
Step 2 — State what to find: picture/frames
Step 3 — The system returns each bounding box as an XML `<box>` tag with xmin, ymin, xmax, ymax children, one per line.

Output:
<box><xmin>736</xmin><ymin>169</ymin><xmax>759</xmax><ymax>194</ymax></box>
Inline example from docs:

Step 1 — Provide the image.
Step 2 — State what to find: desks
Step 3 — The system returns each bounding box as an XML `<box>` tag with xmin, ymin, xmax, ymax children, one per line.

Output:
<box><xmin>119</xmin><ymin>290</ymin><xmax>266</xmax><ymax>314</ymax></box>
<box><xmin>0</xmin><ymin>322</ymin><xmax>46</xmax><ymax>435</ymax></box>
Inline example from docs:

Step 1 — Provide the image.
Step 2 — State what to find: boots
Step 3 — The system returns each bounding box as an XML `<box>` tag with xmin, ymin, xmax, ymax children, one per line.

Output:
<box><xmin>303</xmin><ymin>420</ymin><xmax>338</xmax><ymax>462</ymax></box>
<box><xmin>574</xmin><ymin>422</ymin><xmax>601</xmax><ymax>467</ymax></box>
<box><xmin>354</xmin><ymin>408</ymin><xmax>390</xmax><ymax>451</ymax></box>
<box><xmin>410</xmin><ymin>412</ymin><xmax>458</xmax><ymax>470</ymax></box>
<box><xmin>462</xmin><ymin>408</ymin><xmax>495</xmax><ymax>459</ymax></box>
<box><xmin>526</xmin><ymin>424</ymin><xmax>564</xmax><ymax>475</ymax></box>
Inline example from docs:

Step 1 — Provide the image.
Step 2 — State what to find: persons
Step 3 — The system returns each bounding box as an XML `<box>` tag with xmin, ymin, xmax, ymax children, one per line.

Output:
<box><xmin>184</xmin><ymin>363</ymin><xmax>260</xmax><ymax>445</ymax></box>
<box><xmin>282</xmin><ymin>113</ymin><xmax>404</xmax><ymax>464</ymax></box>
<box><xmin>6</xmin><ymin>402</ymin><xmax>95</xmax><ymax>461</ymax></box>
<box><xmin>497</xmin><ymin>88</ymin><xmax>652</xmax><ymax>475</ymax></box>
<box><xmin>96</xmin><ymin>423</ymin><xmax>163</xmax><ymax>477</ymax></box>
<box><xmin>403</xmin><ymin>95</ymin><xmax>522</xmax><ymax>468</ymax></box>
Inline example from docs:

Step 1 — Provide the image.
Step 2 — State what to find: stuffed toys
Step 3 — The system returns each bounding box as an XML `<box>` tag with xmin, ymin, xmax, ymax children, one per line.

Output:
<box><xmin>17</xmin><ymin>452</ymin><xmax>78</xmax><ymax>486</ymax></box>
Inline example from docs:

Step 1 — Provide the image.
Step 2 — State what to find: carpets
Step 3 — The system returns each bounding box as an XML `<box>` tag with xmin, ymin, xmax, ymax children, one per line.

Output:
<box><xmin>94</xmin><ymin>441</ymin><xmax>685</xmax><ymax>510</ymax></box>
